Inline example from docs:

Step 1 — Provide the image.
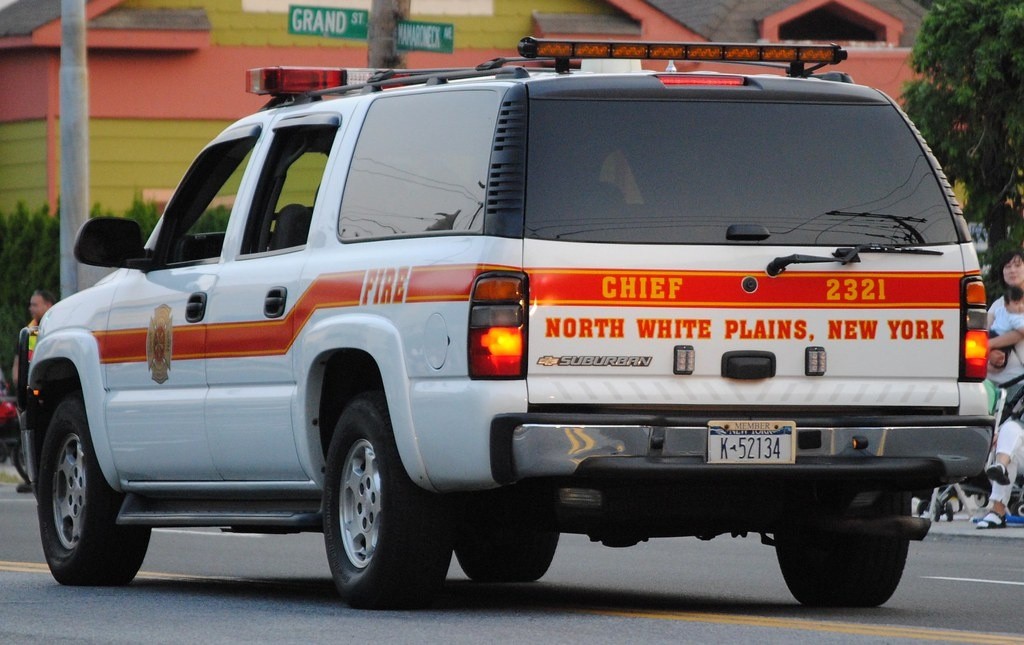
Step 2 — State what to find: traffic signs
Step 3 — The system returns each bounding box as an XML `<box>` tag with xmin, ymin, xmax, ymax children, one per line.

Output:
<box><xmin>286</xmin><ymin>3</ymin><xmax>369</xmax><ymax>41</ymax></box>
<box><xmin>395</xmin><ymin>22</ymin><xmax>453</xmax><ymax>55</ymax></box>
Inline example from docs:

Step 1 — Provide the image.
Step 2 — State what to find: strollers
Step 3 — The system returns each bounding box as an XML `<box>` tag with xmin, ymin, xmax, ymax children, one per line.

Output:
<box><xmin>917</xmin><ymin>370</ymin><xmax>1024</xmax><ymax>528</ymax></box>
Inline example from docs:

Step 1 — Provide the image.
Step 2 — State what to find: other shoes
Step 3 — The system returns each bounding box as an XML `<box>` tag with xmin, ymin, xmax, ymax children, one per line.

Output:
<box><xmin>16</xmin><ymin>482</ymin><xmax>33</xmax><ymax>493</ymax></box>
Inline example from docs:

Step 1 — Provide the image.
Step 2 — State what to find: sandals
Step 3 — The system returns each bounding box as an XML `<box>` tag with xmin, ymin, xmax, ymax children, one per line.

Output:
<box><xmin>976</xmin><ymin>511</ymin><xmax>1008</xmax><ymax>528</ymax></box>
<box><xmin>986</xmin><ymin>462</ymin><xmax>1010</xmax><ymax>485</ymax></box>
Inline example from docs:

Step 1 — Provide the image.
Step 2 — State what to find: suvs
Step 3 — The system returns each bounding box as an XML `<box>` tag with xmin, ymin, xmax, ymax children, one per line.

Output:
<box><xmin>19</xmin><ymin>33</ymin><xmax>1002</xmax><ymax>608</ymax></box>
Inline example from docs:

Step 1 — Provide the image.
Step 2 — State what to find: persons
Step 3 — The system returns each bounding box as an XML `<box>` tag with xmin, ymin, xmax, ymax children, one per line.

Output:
<box><xmin>12</xmin><ymin>289</ymin><xmax>57</xmax><ymax>493</ymax></box>
<box><xmin>976</xmin><ymin>249</ymin><xmax>1023</xmax><ymax>529</ymax></box>
